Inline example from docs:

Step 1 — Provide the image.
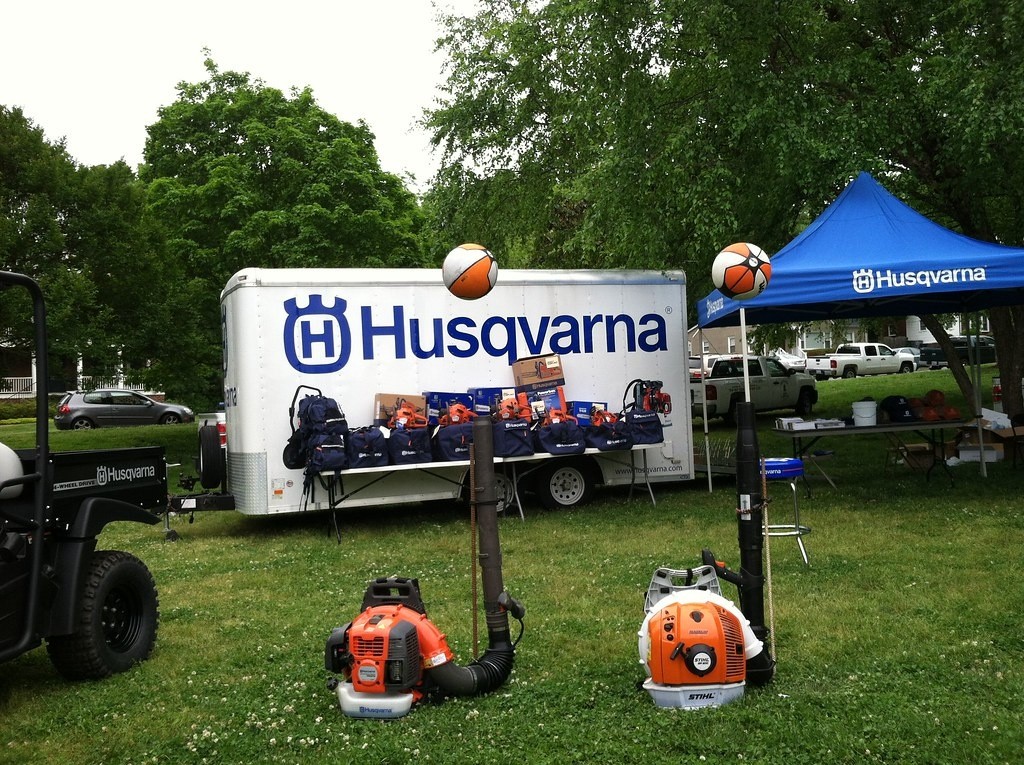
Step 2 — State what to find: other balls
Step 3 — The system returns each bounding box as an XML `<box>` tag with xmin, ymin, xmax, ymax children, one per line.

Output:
<box><xmin>711</xmin><ymin>242</ymin><xmax>774</xmax><ymax>301</ymax></box>
<box><xmin>440</xmin><ymin>241</ymin><xmax>501</xmax><ymax>301</ymax></box>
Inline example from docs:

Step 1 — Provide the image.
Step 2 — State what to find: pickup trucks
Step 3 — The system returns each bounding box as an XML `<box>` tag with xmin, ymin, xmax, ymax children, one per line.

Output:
<box><xmin>920</xmin><ymin>336</ymin><xmax>996</xmax><ymax>370</ymax></box>
<box><xmin>805</xmin><ymin>342</ymin><xmax>918</xmax><ymax>379</ymax></box>
<box><xmin>689</xmin><ymin>355</ymin><xmax>819</xmax><ymax>429</ymax></box>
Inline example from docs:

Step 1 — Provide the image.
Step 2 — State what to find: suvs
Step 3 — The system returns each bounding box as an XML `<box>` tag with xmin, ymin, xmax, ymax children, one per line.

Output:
<box><xmin>53</xmin><ymin>389</ymin><xmax>195</xmax><ymax>430</ymax></box>
<box><xmin>0</xmin><ymin>278</ymin><xmax>169</xmax><ymax>684</ymax></box>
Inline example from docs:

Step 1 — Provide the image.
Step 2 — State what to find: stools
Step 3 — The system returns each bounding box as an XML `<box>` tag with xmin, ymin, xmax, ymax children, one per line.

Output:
<box><xmin>759</xmin><ymin>457</ymin><xmax>813</xmax><ymax>566</ymax></box>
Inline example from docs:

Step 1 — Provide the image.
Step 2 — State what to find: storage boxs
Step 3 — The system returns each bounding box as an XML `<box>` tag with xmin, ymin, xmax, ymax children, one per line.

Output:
<box><xmin>373</xmin><ymin>351</ymin><xmax>608</xmax><ymax>427</ymax></box>
<box><xmin>899</xmin><ymin>407</ymin><xmax>1024</xmax><ymax>469</ymax></box>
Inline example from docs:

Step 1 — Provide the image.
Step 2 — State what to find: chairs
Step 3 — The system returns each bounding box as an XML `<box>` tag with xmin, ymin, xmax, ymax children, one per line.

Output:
<box><xmin>718</xmin><ymin>366</ymin><xmax>730</xmax><ymax>375</ymax></box>
<box><xmin>749</xmin><ymin>363</ymin><xmax>763</xmax><ymax>375</ymax></box>
<box><xmin>882</xmin><ymin>430</ymin><xmax>922</xmax><ymax>472</ymax></box>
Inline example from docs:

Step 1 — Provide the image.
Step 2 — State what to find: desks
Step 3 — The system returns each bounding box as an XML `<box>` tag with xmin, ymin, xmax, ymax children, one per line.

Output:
<box><xmin>504</xmin><ymin>440</ymin><xmax>666</xmax><ymax>521</ymax></box>
<box><xmin>772</xmin><ymin>417</ymin><xmax>973</xmax><ymax>498</ymax></box>
<box><xmin>318</xmin><ymin>454</ymin><xmax>504</xmax><ymax>546</ymax></box>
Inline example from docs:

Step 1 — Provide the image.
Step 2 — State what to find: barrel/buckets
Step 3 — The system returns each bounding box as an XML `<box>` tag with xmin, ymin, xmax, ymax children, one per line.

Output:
<box><xmin>852</xmin><ymin>401</ymin><xmax>876</xmax><ymax>425</ymax></box>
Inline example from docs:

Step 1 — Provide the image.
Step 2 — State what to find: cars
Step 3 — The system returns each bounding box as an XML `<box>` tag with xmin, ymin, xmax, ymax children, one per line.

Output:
<box><xmin>689</xmin><ymin>355</ymin><xmax>721</xmax><ymax>382</ymax></box>
